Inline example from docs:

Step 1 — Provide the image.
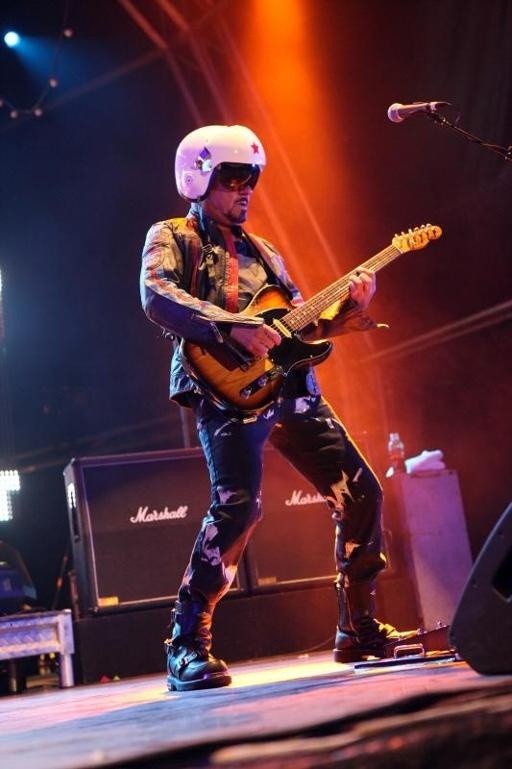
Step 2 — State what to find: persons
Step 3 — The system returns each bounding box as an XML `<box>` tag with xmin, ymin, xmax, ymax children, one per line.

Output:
<box><xmin>140</xmin><ymin>123</ymin><xmax>428</xmax><ymax>692</ymax></box>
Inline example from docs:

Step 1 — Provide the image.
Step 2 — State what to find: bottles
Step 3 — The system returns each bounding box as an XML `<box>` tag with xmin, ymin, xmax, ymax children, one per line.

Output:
<box><xmin>387</xmin><ymin>431</ymin><xmax>406</xmax><ymax>475</ymax></box>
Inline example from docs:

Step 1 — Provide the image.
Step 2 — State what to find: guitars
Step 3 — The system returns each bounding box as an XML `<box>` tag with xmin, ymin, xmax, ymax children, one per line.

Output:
<box><xmin>178</xmin><ymin>222</ymin><xmax>444</xmax><ymax>416</ymax></box>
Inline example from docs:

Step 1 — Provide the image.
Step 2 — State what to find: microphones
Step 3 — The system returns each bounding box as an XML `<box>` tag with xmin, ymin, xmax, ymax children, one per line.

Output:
<box><xmin>388</xmin><ymin>102</ymin><xmax>451</xmax><ymax>123</ymax></box>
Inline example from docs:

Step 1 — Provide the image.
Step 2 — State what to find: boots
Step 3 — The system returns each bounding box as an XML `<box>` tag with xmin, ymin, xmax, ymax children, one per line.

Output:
<box><xmin>333</xmin><ymin>581</ymin><xmax>422</xmax><ymax>663</ymax></box>
<box><xmin>163</xmin><ymin>602</ymin><xmax>231</xmax><ymax>692</ymax></box>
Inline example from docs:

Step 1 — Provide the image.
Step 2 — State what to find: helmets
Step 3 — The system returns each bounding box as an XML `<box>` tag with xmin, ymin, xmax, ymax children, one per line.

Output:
<box><xmin>174</xmin><ymin>123</ymin><xmax>267</xmax><ymax>203</ymax></box>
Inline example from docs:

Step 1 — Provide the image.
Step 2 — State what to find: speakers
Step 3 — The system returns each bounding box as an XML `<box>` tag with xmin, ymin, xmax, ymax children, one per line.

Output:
<box><xmin>62</xmin><ymin>446</ymin><xmax>249</xmax><ymax>615</ymax></box>
<box><xmin>449</xmin><ymin>502</ymin><xmax>511</xmax><ymax>676</ymax></box>
<box><xmin>245</xmin><ymin>436</ymin><xmax>389</xmax><ymax>590</ymax></box>
